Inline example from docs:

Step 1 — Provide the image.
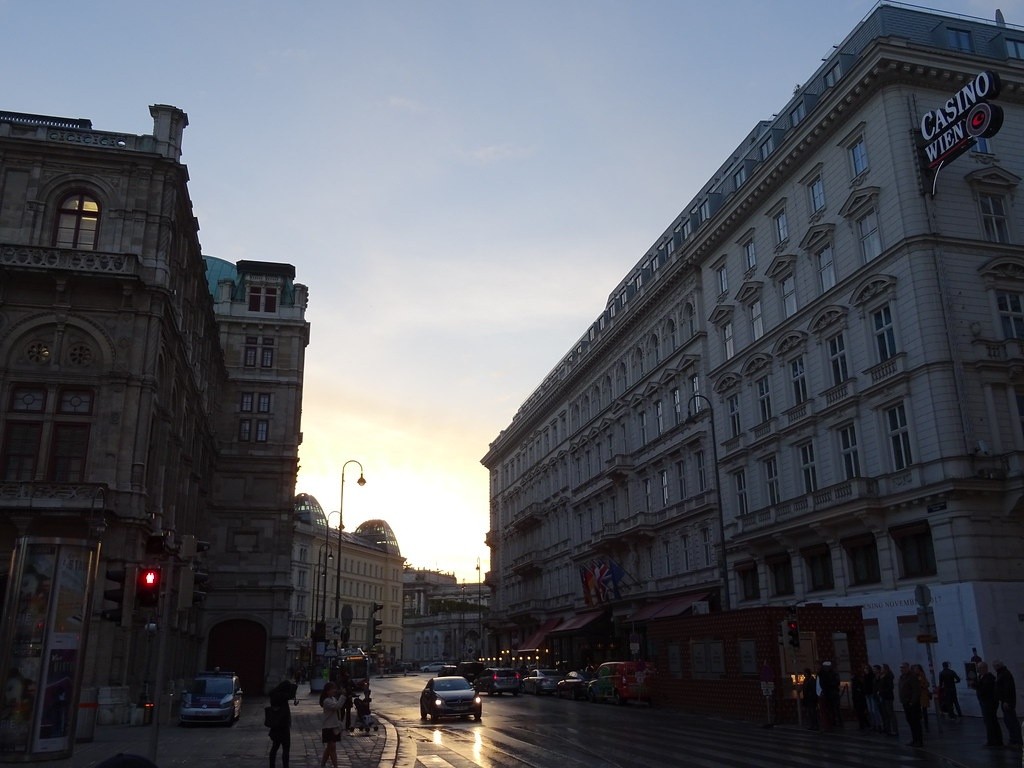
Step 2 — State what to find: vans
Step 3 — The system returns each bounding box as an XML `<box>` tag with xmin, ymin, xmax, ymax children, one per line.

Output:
<box><xmin>586</xmin><ymin>661</ymin><xmax>656</xmax><ymax>706</ymax></box>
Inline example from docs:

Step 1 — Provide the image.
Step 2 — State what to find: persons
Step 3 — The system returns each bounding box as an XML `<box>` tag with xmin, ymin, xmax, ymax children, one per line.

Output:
<box><xmin>290</xmin><ymin>663</ymin><xmax>316</xmax><ymax>684</ymax></box>
<box><xmin>939</xmin><ymin>661</ymin><xmax>962</xmax><ymax>718</ymax></box>
<box><xmin>850</xmin><ymin>664</ymin><xmax>897</xmax><ymax>736</ymax></box>
<box><xmin>796</xmin><ymin>662</ymin><xmax>844</xmax><ymax>729</ymax></box>
<box><xmin>971</xmin><ymin>659</ymin><xmax>1022</xmax><ymax>747</ymax></box>
<box><xmin>338</xmin><ymin>670</ymin><xmax>355</xmax><ymax>729</ymax></box>
<box><xmin>898</xmin><ymin>662</ymin><xmax>930</xmax><ymax>748</ymax></box>
<box><xmin>269</xmin><ymin>680</ymin><xmax>298</xmax><ymax>768</ymax></box>
<box><xmin>214</xmin><ymin>665</ymin><xmax>225</xmax><ymax>672</ymax></box>
<box><xmin>319</xmin><ymin>683</ymin><xmax>346</xmax><ymax>768</ymax></box>
<box><xmin>585</xmin><ymin>656</ymin><xmax>616</xmax><ymax>672</ymax></box>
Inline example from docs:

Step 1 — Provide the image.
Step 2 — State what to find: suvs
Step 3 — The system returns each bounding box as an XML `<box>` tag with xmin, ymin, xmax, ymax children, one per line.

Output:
<box><xmin>454</xmin><ymin>662</ymin><xmax>485</xmax><ymax>682</ymax></box>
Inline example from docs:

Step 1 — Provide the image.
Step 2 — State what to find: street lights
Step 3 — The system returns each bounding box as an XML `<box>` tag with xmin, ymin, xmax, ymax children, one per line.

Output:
<box><xmin>322</xmin><ymin>507</ymin><xmax>344</xmax><ymax>638</ymax></box>
<box><xmin>460</xmin><ymin>577</ymin><xmax>467</xmax><ymax>661</ymax></box>
<box><xmin>684</xmin><ymin>395</ymin><xmax>732</xmax><ymax>612</ymax></box>
<box><xmin>310</xmin><ymin>543</ymin><xmax>333</xmax><ymax>684</ymax></box>
<box><xmin>475</xmin><ymin>556</ymin><xmax>482</xmax><ymax>661</ymax></box>
<box><xmin>333</xmin><ymin>460</ymin><xmax>366</xmax><ymax>679</ymax></box>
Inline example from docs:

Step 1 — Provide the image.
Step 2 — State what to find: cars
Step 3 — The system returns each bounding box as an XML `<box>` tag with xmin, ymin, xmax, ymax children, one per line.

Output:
<box><xmin>419</xmin><ymin>661</ymin><xmax>447</xmax><ymax>673</ymax></box>
<box><xmin>421</xmin><ymin>676</ymin><xmax>482</xmax><ymax>722</ymax></box>
<box><xmin>374</xmin><ymin>665</ymin><xmax>394</xmax><ymax>673</ymax></box>
<box><xmin>522</xmin><ymin>668</ymin><xmax>564</xmax><ymax>695</ymax></box>
<box><xmin>438</xmin><ymin>665</ymin><xmax>456</xmax><ymax>676</ymax></box>
<box><xmin>473</xmin><ymin>668</ymin><xmax>521</xmax><ymax>696</ymax></box>
<box><xmin>557</xmin><ymin>670</ymin><xmax>593</xmax><ymax>700</ymax></box>
<box><xmin>178</xmin><ymin>674</ymin><xmax>244</xmax><ymax>727</ymax></box>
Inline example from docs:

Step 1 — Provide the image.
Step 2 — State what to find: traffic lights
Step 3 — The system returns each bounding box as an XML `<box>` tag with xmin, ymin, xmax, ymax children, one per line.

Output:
<box><xmin>371</xmin><ymin>617</ymin><xmax>383</xmax><ymax>645</ymax></box>
<box><xmin>370</xmin><ymin>603</ymin><xmax>384</xmax><ymax>613</ymax></box>
<box><xmin>177</xmin><ymin>534</ymin><xmax>209</xmax><ymax>610</ymax></box>
<box><xmin>101</xmin><ymin>566</ymin><xmax>125</xmax><ymax>626</ymax></box>
<box><xmin>787</xmin><ymin>621</ymin><xmax>799</xmax><ymax>648</ymax></box>
<box><xmin>141</xmin><ymin>568</ymin><xmax>161</xmax><ymax>596</ymax></box>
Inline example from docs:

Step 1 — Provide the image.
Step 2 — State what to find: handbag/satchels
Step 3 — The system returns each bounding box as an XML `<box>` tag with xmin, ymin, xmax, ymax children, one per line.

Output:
<box><xmin>333</xmin><ymin>720</ymin><xmax>344</xmax><ymax>735</ymax></box>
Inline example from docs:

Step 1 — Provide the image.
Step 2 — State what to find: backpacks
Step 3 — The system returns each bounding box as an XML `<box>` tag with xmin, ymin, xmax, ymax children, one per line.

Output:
<box><xmin>264</xmin><ymin>707</ymin><xmax>275</xmax><ymax>727</ymax></box>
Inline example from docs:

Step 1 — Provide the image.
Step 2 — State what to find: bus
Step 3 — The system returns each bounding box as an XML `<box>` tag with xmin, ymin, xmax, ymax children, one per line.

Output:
<box><xmin>337</xmin><ymin>655</ymin><xmax>370</xmax><ymax>691</ymax></box>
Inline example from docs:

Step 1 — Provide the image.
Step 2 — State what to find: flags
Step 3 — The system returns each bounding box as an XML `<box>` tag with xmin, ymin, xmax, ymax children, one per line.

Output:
<box><xmin>580</xmin><ymin>558</ymin><xmax>625</xmax><ymax>607</ymax></box>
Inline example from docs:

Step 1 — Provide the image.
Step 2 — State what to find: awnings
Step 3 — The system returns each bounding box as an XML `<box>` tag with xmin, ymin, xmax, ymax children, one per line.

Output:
<box><xmin>549</xmin><ymin>611</ymin><xmax>608</xmax><ymax>635</ymax></box>
<box><xmin>518</xmin><ymin>618</ymin><xmax>561</xmax><ymax>651</ymax></box>
<box><xmin>623</xmin><ymin>591</ymin><xmax>710</xmax><ymax>621</ymax></box>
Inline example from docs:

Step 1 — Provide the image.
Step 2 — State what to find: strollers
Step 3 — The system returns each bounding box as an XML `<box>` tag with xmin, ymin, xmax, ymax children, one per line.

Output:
<box><xmin>348</xmin><ymin>695</ymin><xmax>379</xmax><ymax>732</ymax></box>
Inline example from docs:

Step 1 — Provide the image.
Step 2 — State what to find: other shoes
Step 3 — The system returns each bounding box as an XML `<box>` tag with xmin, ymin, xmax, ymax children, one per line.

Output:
<box><xmin>905</xmin><ymin>742</ymin><xmax>924</xmax><ymax>747</ymax></box>
<box><xmin>346</xmin><ymin>730</ymin><xmax>350</xmax><ymax>735</ymax></box>
